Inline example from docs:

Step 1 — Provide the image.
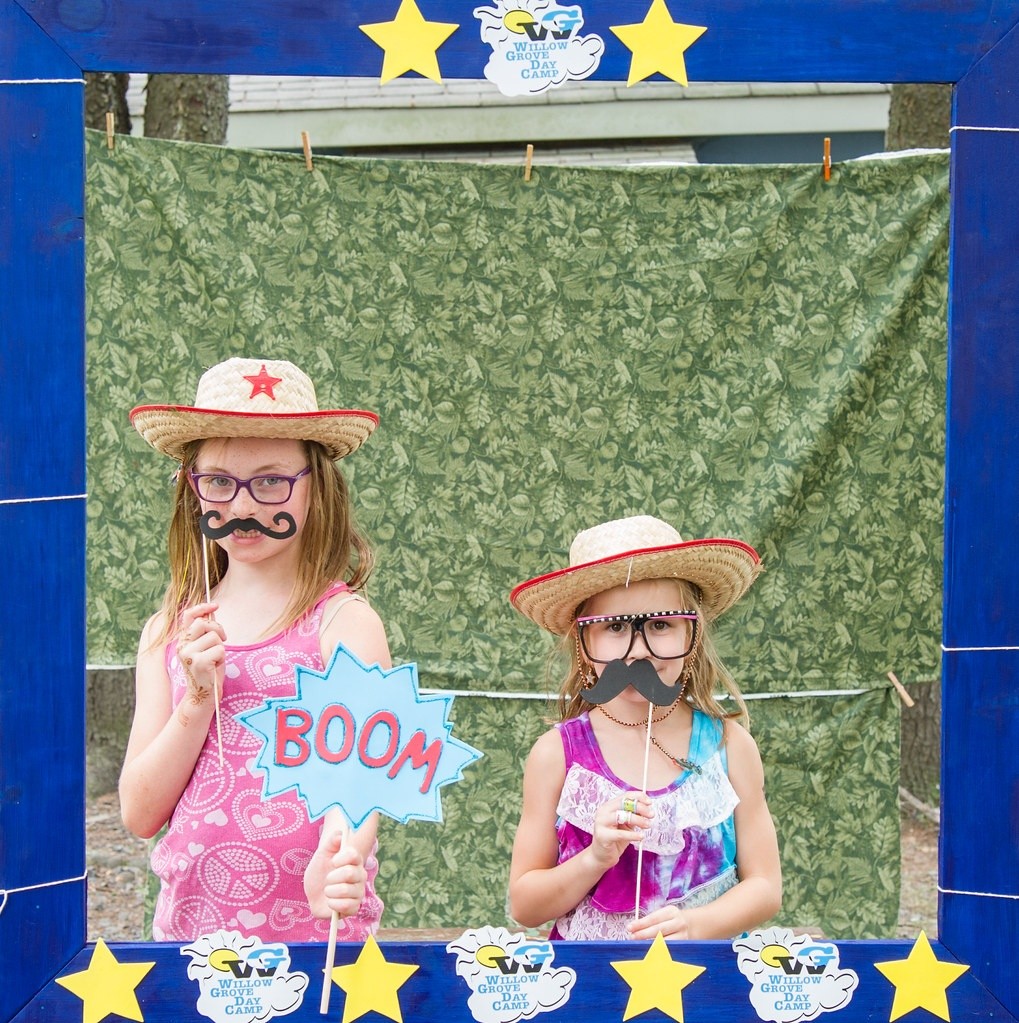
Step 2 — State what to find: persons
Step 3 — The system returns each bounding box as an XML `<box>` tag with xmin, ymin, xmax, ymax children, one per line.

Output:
<box><xmin>507</xmin><ymin>515</ymin><xmax>782</xmax><ymax>942</ymax></box>
<box><xmin>118</xmin><ymin>358</ymin><xmax>392</xmax><ymax>944</ymax></box>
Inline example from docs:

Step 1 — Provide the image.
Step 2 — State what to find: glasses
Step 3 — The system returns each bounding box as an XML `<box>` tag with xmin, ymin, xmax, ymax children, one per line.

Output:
<box><xmin>187</xmin><ymin>465</ymin><xmax>312</xmax><ymax>505</ymax></box>
<box><xmin>575</xmin><ymin>615</ymin><xmax>700</xmax><ymax>664</ymax></box>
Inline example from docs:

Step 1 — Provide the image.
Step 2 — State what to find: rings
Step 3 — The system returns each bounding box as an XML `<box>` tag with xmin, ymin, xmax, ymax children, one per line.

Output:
<box><xmin>623</xmin><ymin>797</ymin><xmax>638</xmax><ymax>812</ymax></box>
<box><xmin>616</xmin><ymin>811</ymin><xmax>632</xmax><ymax>826</ymax></box>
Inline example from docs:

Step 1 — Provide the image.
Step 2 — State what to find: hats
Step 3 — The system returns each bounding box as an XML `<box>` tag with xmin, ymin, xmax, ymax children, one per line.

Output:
<box><xmin>507</xmin><ymin>513</ymin><xmax>762</xmax><ymax>642</ymax></box>
<box><xmin>127</xmin><ymin>355</ymin><xmax>381</xmax><ymax>466</ymax></box>
